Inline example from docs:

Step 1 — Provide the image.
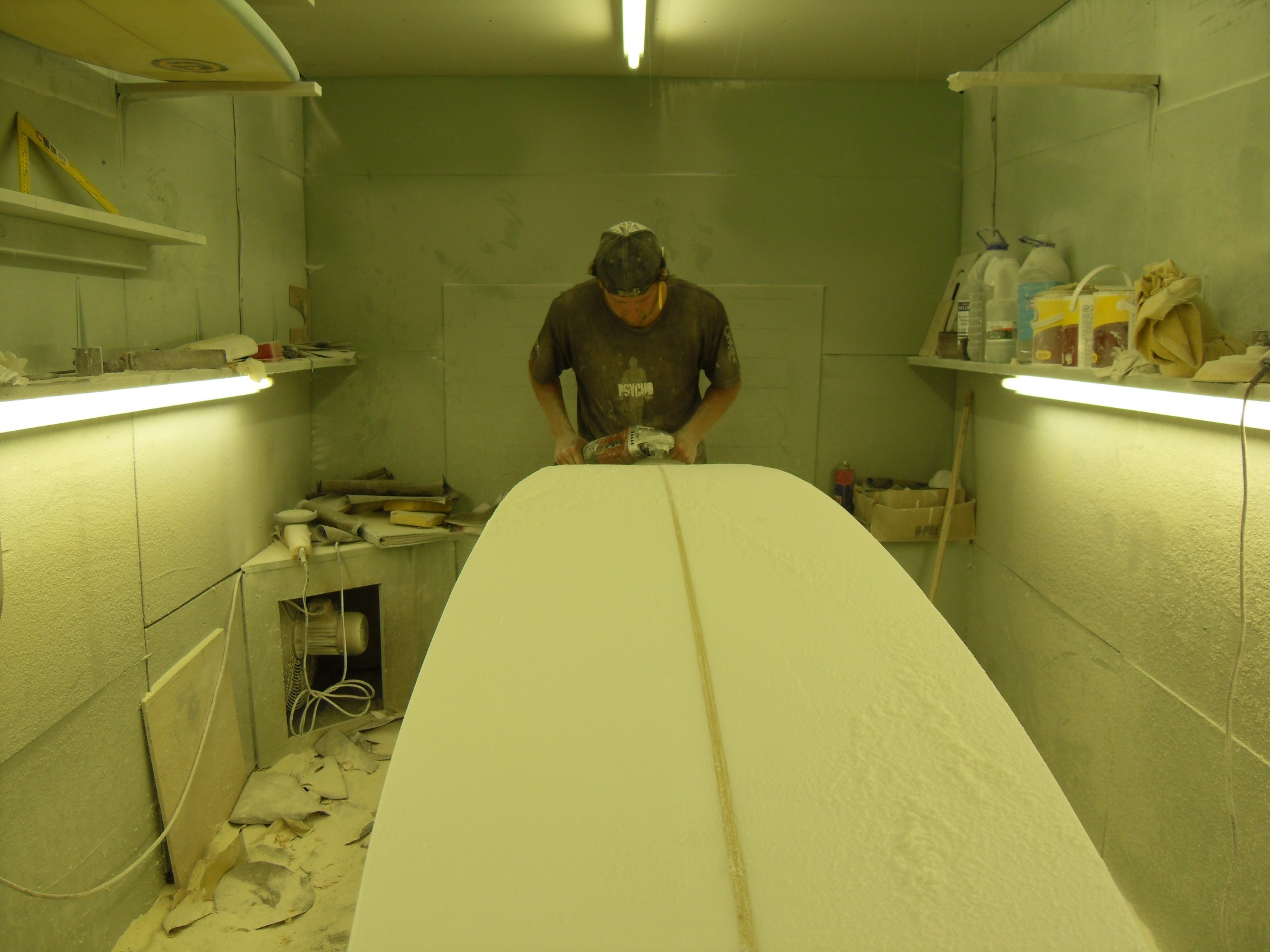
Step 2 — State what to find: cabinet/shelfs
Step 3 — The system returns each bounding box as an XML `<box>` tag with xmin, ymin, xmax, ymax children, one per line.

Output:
<box><xmin>0</xmin><ymin>1</ymin><xmax>355</xmax><ymax>401</ymax></box>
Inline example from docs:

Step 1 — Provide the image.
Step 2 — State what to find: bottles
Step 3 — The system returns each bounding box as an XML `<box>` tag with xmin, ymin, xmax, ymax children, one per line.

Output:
<box><xmin>1017</xmin><ymin>234</ymin><xmax>1070</xmax><ymax>364</ymax></box>
<box><xmin>985</xmin><ymin>258</ymin><xmax>1020</xmax><ymax>363</ymax></box>
<box><xmin>957</xmin><ymin>283</ymin><xmax>970</xmax><ymax>360</ymax></box>
<box><xmin>835</xmin><ymin>460</ymin><xmax>855</xmax><ymax>516</ymax></box>
<box><xmin>967</xmin><ymin>227</ymin><xmax>1010</xmax><ymax>362</ymax></box>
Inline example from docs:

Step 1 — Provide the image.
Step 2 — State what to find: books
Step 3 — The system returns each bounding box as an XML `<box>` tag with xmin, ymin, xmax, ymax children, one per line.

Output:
<box><xmin>349</xmin><ymin>510</ymin><xmax>452</xmax><ymax>549</ymax></box>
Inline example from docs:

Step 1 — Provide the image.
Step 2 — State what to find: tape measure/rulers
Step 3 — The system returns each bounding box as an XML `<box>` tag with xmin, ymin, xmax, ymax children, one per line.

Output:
<box><xmin>17</xmin><ymin>110</ymin><xmax>122</xmax><ymax>217</ymax></box>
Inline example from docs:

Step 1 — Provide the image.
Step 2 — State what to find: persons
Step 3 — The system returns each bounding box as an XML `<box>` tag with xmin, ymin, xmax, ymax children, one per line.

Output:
<box><xmin>528</xmin><ymin>221</ymin><xmax>742</xmax><ymax>465</ymax></box>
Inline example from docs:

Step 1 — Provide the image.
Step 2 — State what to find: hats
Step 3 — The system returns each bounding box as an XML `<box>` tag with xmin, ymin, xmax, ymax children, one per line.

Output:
<box><xmin>595</xmin><ymin>222</ymin><xmax>662</xmax><ymax>296</ymax></box>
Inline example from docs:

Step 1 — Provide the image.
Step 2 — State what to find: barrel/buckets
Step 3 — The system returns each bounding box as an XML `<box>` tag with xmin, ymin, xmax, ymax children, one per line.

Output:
<box><xmin>1091</xmin><ymin>291</ymin><xmax>1138</xmax><ymax>369</ymax></box>
<box><xmin>1033</xmin><ymin>296</ymin><xmax>1064</xmax><ymax>364</ymax></box>
<box><xmin>1094</xmin><ymin>286</ymin><xmax>1136</xmax><ymax>291</ymax></box>
<box><xmin>1061</xmin><ymin>295</ymin><xmax>1094</xmax><ymax>370</ymax></box>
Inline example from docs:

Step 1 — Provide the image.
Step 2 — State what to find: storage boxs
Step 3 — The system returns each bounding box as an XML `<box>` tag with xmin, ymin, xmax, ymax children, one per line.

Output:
<box><xmin>853</xmin><ymin>483</ymin><xmax>976</xmax><ymax>542</ymax></box>
